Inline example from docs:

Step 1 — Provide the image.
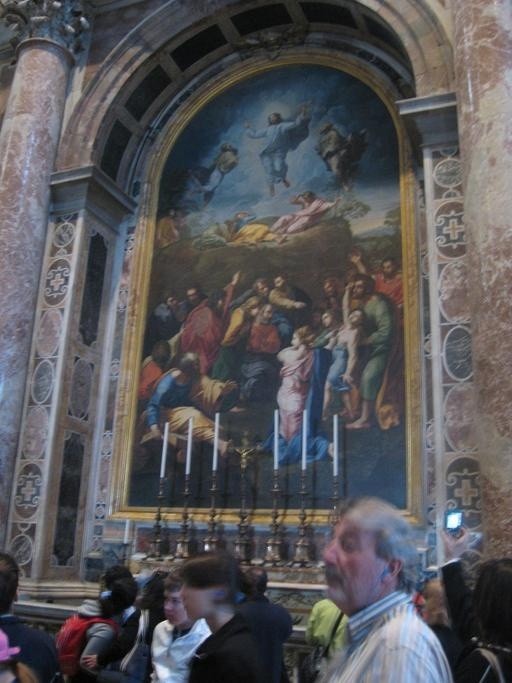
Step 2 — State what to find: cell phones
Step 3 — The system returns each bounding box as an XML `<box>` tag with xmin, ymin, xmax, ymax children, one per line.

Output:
<box><xmin>445</xmin><ymin>509</ymin><xmax>463</xmax><ymax>534</ymax></box>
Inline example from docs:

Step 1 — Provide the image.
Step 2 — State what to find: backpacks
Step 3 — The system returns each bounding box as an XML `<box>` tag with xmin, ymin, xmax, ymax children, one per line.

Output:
<box><xmin>54</xmin><ymin>610</ymin><xmax>123</xmax><ymax>677</ymax></box>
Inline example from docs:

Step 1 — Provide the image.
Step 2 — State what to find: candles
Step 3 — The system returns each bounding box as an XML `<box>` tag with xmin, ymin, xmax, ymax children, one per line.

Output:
<box><xmin>122</xmin><ymin>407</ymin><xmax>340</xmax><ymax>545</ymax></box>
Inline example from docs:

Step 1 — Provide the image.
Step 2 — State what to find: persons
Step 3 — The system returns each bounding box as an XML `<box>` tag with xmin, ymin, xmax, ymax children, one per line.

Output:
<box><xmin>243</xmin><ymin>103</ymin><xmax>312</xmax><ymax>196</ymax></box>
<box><xmin>314</xmin><ymin>121</ymin><xmax>369</xmax><ymax>192</ymax></box>
<box><xmin>186</xmin><ymin>142</ymin><xmax>240</xmax><ymax>194</ymax></box>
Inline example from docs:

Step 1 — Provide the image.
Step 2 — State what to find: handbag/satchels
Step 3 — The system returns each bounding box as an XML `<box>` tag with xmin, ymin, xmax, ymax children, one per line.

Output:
<box><xmin>101</xmin><ymin>641</ymin><xmax>152</xmax><ymax>682</ymax></box>
<box><xmin>297</xmin><ymin>642</ymin><xmax>330</xmax><ymax>682</ymax></box>
